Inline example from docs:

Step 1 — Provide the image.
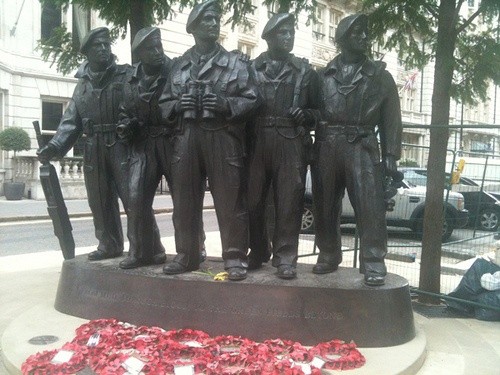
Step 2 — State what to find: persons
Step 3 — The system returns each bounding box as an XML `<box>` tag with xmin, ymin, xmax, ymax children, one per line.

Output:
<box><xmin>313</xmin><ymin>13</ymin><xmax>402</xmax><ymax>288</ymax></box>
<box><xmin>36</xmin><ymin>27</ymin><xmax>167</xmax><ymax>265</ymax></box>
<box><xmin>157</xmin><ymin>0</ymin><xmax>267</xmax><ymax>281</ymax></box>
<box><xmin>171</xmin><ymin>11</ymin><xmax>325</xmax><ymax>280</ymax></box>
<box><xmin>116</xmin><ymin>26</ymin><xmax>250</xmax><ymax>269</ymax></box>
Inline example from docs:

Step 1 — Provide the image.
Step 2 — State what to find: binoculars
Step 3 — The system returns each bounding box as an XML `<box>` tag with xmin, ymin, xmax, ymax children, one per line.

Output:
<box><xmin>185</xmin><ymin>79</ymin><xmax>215</xmax><ymax>120</ymax></box>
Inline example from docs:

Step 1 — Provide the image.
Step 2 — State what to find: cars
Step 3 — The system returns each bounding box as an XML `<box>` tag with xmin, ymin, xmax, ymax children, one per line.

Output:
<box><xmin>298</xmin><ymin>164</ymin><xmax>500</xmax><ymax>243</ymax></box>
